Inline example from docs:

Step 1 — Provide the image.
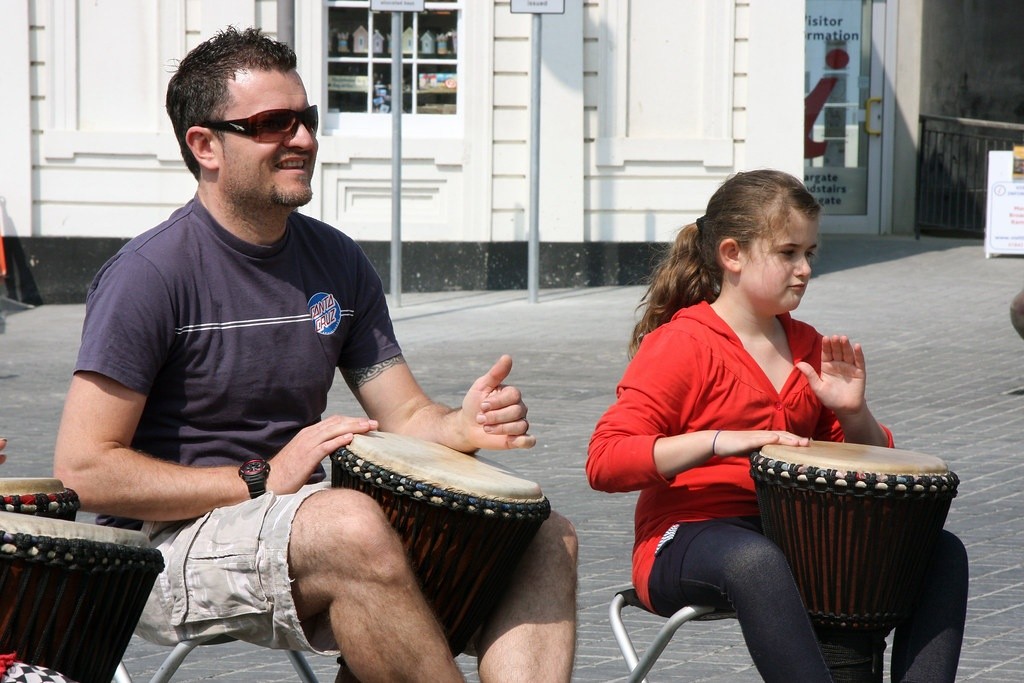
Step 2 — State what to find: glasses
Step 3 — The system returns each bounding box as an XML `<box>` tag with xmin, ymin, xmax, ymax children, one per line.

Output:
<box><xmin>199</xmin><ymin>103</ymin><xmax>318</xmax><ymax>143</ymax></box>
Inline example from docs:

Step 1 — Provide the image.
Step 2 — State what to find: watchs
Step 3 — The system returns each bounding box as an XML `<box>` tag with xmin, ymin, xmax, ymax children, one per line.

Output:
<box><xmin>238</xmin><ymin>458</ymin><xmax>271</xmax><ymax>499</ymax></box>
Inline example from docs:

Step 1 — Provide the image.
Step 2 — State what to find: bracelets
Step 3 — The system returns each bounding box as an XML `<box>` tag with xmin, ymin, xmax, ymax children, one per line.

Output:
<box><xmin>712</xmin><ymin>430</ymin><xmax>721</xmax><ymax>455</ymax></box>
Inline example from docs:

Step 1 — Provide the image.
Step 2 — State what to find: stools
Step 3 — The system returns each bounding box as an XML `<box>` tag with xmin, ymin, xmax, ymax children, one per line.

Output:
<box><xmin>147</xmin><ymin>635</ymin><xmax>322</xmax><ymax>683</ymax></box>
<box><xmin>608</xmin><ymin>584</ymin><xmax>740</xmax><ymax>683</ymax></box>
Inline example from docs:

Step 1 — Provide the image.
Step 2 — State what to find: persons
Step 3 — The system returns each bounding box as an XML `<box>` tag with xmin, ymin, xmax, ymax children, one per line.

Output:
<box><xmin>584</xmin><ymin>171</ymin><xmax>970</xmax><ymax>683</ymax></box>
<box><xmin>53</xmin><ymin>27</ymin><xmax>579</xmax><ymax>683</ymax></box>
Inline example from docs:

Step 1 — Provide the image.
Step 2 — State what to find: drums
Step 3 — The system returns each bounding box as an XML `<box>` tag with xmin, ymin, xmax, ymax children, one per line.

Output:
<box><xmin>1</xmin><ymin>472</ymin><xmax>166</xmax><ymax>683</ymax></box>
<box><xmin>331</xmin><ymin>427</ymin><xmax>552</xmax><ymax>682</ymax></box>
<box><xmin>748</xmin><ymin>440</ymin><xmax>962</xmax><ymax>683</ymax></box>
